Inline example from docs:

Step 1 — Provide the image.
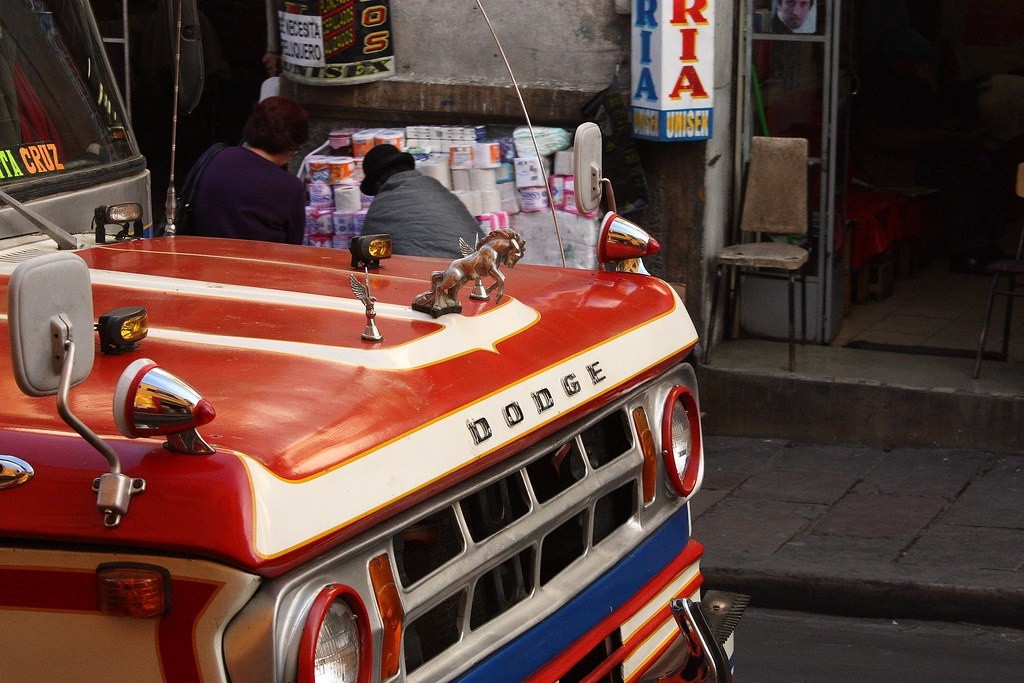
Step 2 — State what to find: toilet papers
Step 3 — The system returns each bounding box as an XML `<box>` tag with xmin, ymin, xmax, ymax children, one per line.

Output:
<box><xmin>305</xmin><ymin>126</ymin><xmax>605</xmax><ymax>270</ymax></box>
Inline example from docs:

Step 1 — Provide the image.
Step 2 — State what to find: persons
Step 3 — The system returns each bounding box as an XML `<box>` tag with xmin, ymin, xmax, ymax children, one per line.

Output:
<box><xmin>360</xmin><ymin>144</ymin><xmax>486</xmax><ymax>260</ymax></box>
<box><xmin>179</xmin><ymin>97</ymin><xmax>309</xmax><ymax>245</ymax></box>
<box><xmin>771</xmin><ymin>0</ymin><xmax>813</xmax><ymax>34</ymax></box>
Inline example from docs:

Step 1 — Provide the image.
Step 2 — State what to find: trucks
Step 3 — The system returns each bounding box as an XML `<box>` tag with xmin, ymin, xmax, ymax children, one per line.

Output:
<box><xmin>0</xmin><ymin>121</ymin><xmax>753</xmax><ymax>683</ymax></box>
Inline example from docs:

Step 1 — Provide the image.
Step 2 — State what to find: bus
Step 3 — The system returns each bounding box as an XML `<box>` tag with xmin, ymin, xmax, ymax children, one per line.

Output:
<box><xmin>0</xmin><ymin>0</ymin><xmax>207</xmax><ymax>247</ymax></box>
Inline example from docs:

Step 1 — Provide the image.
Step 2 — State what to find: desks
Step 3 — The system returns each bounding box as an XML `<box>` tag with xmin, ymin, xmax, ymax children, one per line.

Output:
<box><xmin>829</xmin><ymin>182</ymin><xmax>945</xmax><ymax>317</ymax></box>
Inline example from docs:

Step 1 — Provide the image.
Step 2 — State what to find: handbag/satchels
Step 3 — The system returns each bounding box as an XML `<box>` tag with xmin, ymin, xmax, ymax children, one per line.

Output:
<box><xmin>154</xmin><ymin>144</ymin><xmax>227</xmax><ymax>237</ymax></box>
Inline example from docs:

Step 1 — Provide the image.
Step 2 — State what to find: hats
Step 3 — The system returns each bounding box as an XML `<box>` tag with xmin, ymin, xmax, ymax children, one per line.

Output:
<box><xmin>360</xmin><ymin>144</ymin><xmax>415</xmax><ymax>195</ymax></box>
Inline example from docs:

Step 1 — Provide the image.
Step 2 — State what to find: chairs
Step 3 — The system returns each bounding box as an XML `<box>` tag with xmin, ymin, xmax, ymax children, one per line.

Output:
<box><xmin>970</xmin><ymin>162</ymin><xmax>1024</xmax><ymax>379</ymax></box>
<box><xmin>704</xmin><ymin>135</ymin><xmax>815</xmax><ymax>371</ymax></box>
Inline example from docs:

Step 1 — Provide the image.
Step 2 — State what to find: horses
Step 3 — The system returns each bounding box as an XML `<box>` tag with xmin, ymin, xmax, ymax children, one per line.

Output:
<box><xmin>429</xmin><ymin>229</ymin><xmax>527</xmax><ymax>318</ymax></box>
